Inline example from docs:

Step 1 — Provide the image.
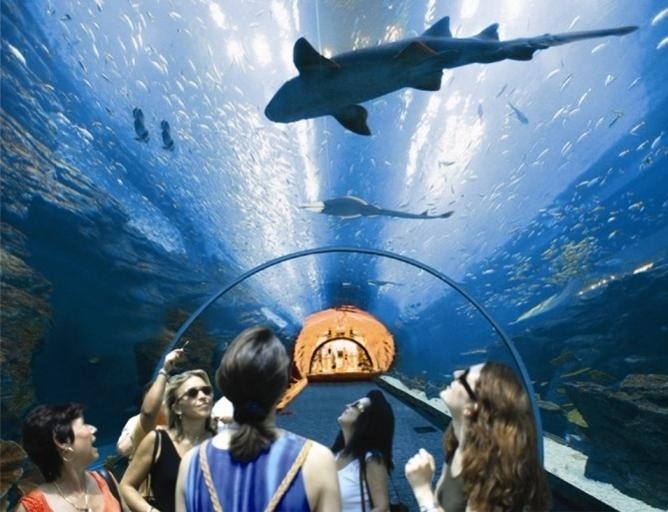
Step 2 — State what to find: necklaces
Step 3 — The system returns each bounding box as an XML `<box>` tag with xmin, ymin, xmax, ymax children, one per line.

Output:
<box><xmin>53</xmin><ymin>473</ymin><xmax>93</xmax><ymax>512</ymax></box>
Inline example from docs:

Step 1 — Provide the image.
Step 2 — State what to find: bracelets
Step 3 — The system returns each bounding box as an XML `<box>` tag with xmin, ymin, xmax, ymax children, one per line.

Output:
<box><xmin>150</xmin><ymin>507</ymin><xmax>155</xmax><ymax>511</ymax></box>
<box><xmin>159</xmin><ymin>368</ymin><xmax>170</xmax><ymax>379</ymax></box>
<box><xmin>418</xmin><ymin>500</ymin><xmax>440</xmax><ymax>512</ymax></box>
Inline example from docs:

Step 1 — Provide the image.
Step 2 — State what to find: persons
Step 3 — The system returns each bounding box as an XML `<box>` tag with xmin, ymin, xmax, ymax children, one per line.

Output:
<box><xmin>128</xmin><ymin>346</ymin><xmax>185</xmax><ymax>502</ymax></box>
<box><xmin>174</xmin><ymin>326</ymin><xmax>343</xmax><ymax>512</ymax></box>
<box><xmin>403</xmin><ymin>360</ymin><xmax>553</xmax><ymax>512</ymax></box>
<box><xmin>115</xmin><ymin>381</ymin><xmax>155</xmax><ymax>458</ymax></box>
<box><xmin>330</xmin><ymin>389</ymin><xmax>396</xmax><ymax>511</ymax></box>
<box><xmin>8</xmin><ymin>401</ymin><xmax>134</xmax><ymax>512</ymax></box>
<box><xmin>118</xmin><ymin>368</ymin><xmax>234</xmax><ymax>512</ymax></box>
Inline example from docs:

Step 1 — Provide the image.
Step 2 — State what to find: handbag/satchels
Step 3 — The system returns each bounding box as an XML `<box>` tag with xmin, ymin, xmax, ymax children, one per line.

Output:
<box><xmin>139</xmin><ymin>478</ymin><xmax>155</xmax><ymax>499</ymax></box>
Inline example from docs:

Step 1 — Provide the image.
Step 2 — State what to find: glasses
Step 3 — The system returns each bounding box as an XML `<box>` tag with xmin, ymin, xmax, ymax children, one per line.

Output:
<box><xmin>458</xmin><ymin>368</ymin><xmax>477</xmax><ymax>401</ymax></box>
<box><xmin>177</xmin><ymin>385</ymin><xmax>212</xmax><ymax>402</ymax></box>
<box><xmin>351</xmin><ymin>400</ymin><xmax>364</xmax><ymax>414</ymax></box>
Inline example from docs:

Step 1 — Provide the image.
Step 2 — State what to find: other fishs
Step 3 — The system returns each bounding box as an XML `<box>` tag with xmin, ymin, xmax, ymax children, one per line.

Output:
<box><xmin>2</xmin><ymin>5</ymin><xmax>346</xmax><ymax>415</ymax></box>
<box><xmin>347</xmin><ymin>6</ymin><xmax>667</xmax><ymax>417</ymax></box>
